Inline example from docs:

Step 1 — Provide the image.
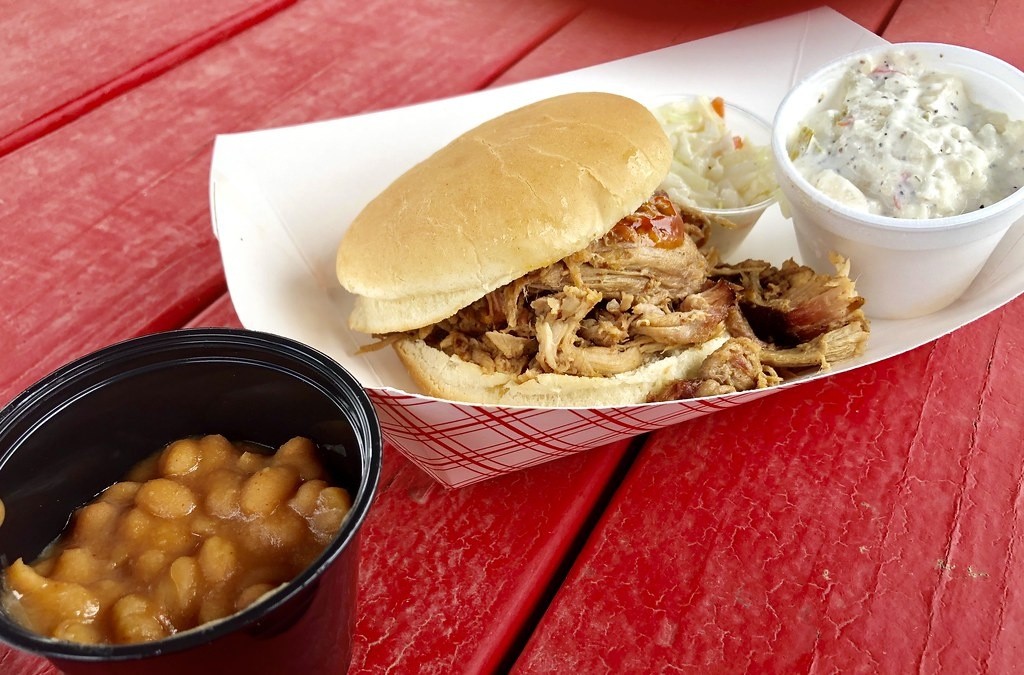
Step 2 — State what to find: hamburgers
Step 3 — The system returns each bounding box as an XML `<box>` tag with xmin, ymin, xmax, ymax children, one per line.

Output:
<box><xmin>335</xmin><ymin>91</ymin><xmax>735</xmax><ymax>406</ymax></box>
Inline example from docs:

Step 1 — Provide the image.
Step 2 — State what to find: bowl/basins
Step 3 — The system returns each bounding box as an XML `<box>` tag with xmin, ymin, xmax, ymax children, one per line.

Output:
<box><xmin>772</xmin><ymin>41</ymin><xmax>1024</xmax><ymax>320</ymax></box>
<box><xmin>0</xmin><ymin>327</ymin><xmax>382</xmax><ymax>662</ymax></box>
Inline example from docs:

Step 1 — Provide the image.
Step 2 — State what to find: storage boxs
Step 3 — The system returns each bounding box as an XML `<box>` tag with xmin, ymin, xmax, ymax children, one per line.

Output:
<box><xmin>209</xmin><ymin>5</ymin><xmax>1024</xmax><ymax>490</ymax></box>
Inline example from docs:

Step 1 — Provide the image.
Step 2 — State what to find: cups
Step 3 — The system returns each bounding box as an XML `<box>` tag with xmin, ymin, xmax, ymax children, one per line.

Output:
<box><xmin>654</xmin><ymin>101</ymin><xmax>782</xmax><ymax>264</ymax></box>
